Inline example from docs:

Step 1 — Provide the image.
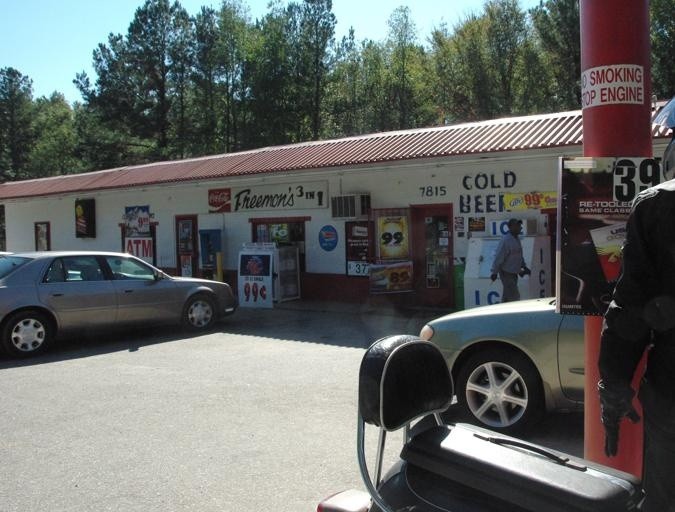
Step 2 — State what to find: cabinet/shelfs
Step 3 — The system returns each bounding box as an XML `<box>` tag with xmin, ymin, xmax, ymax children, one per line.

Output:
<box><xmin>245</xmin><ymin>248</ymin><xmax>300</xmax><ymax>303</ymax></box>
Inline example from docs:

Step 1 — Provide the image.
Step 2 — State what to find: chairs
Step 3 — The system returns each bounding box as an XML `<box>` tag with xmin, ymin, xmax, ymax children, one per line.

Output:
<box><xmin>48</xmin><ymin>269</ymin><xmax>70</xmax><ymax>283</ymax></box>
<box><xmin>82</xmin><ymin>268</ymin><xmax>102</xmax><ymax>280</ymax></box>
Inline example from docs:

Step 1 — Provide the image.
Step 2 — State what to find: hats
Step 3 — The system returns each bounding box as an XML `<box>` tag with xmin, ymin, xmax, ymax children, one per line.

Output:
<box><xmin>507</xmin><ymin>218</ymin><xmax>523</xmax><ymax>227</ymax></box>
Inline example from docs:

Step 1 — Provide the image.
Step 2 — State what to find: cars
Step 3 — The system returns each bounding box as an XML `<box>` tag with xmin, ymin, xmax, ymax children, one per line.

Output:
<box><xmin>0</xmin><ymin>250</ymin><xmax>239</xmax><ymax>362</ymax></box>
<box><xmin>418</xmin><ymin>296</ymin><xmax>585</xmax><ymax>438</ymax></box>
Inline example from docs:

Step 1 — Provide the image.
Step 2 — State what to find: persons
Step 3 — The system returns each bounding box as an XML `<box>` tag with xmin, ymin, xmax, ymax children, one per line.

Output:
<box><xmin>490</xmin><ymin>218</ymin><xmax>532</xmax><ymax>304</ymax></box>
<box><xmin>596</xmin><ymin>101</ymin><xmax>674</xmax><ymax>512</ymax></box>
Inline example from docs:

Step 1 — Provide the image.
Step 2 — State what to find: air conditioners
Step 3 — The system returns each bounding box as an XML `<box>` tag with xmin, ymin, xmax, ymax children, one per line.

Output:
<box><xmin>330</xmin><ymin>192</ymin><xmax>371</xmax><ymax>221</ymax></box>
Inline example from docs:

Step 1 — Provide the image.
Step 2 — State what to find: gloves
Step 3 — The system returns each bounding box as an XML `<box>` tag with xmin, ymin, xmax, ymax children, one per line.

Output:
<box><xmin>597</xmin><ymin>380</ymin><xmax>640</xmax><ymax>457</ymax></box>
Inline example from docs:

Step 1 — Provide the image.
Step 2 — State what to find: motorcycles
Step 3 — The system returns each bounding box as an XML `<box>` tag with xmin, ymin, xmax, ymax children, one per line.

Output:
<box><xmin>315</xmin><ymin>333</ymin><xmax>648</xmax><ymax>512</ymax></box>
